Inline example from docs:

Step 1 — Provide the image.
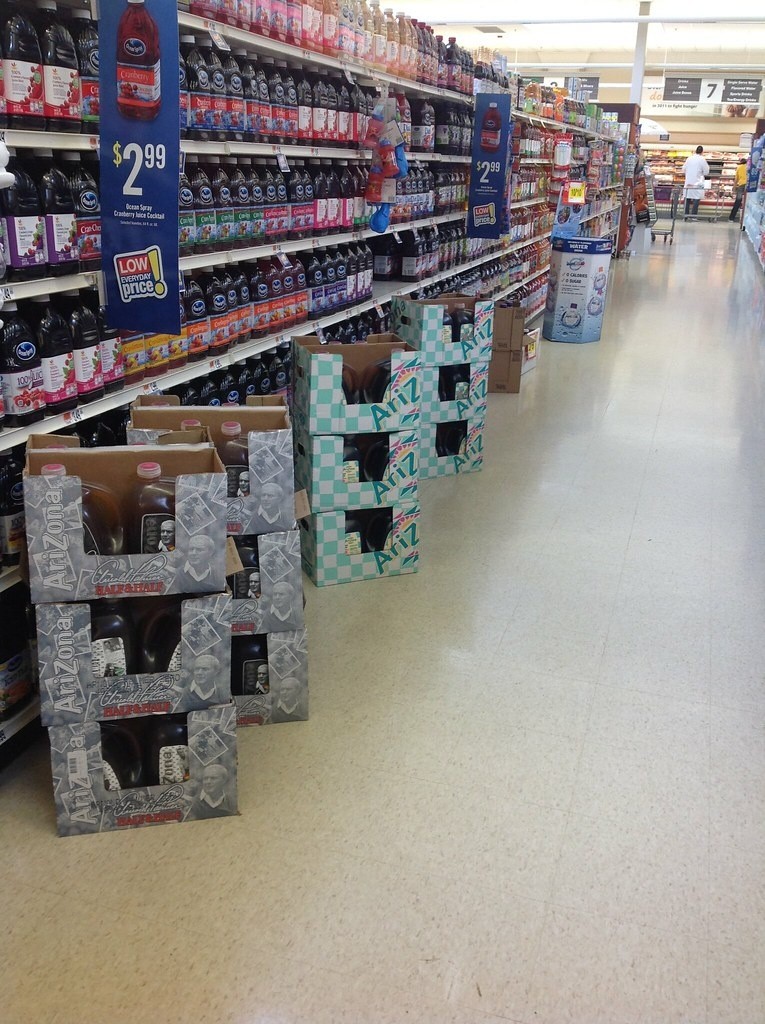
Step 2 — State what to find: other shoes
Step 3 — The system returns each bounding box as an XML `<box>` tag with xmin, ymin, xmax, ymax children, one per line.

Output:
<box><xmin>728</xmin><ymin>219</ymin><xmax>734</xmax><ymax>223</ymax></box>
<box><xmin>682</xmin><ymin>218</ymin><xmax>690</xmax><ymax>221</ymax></box>
<box><xmin>691</xmin><ymin>217</ymin><xmax>697</xmax><ymax>221</ymax></box>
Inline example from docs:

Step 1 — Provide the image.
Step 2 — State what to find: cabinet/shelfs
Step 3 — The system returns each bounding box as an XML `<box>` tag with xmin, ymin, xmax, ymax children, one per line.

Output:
<box><xmin>646</xmin><ymin>156</ymin><xmax>740</xmax><ymax>186</ymax></box>
<box><xmin>0</xmin><ymin>0</ymin><xmax>631</xmax><ymax>746</ymax></box>
<box><xmin>588</xmin><ymin>103</ymin><xmax>640</xmax><ymax>256</ymax></box>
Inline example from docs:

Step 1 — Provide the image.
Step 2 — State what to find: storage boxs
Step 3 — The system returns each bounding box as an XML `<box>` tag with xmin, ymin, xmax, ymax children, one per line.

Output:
<box><xmin>22</xmin><ymin>293</ymin><xmax>526</xmax><ymax>837</ymax></box>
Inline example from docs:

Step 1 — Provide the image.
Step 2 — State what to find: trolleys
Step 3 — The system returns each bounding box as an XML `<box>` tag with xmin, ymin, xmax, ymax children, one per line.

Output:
<box><xmin>683</xmin><ymin>182</ymin><xmax>727</xmax><ymax>223</ymax></box>
<box><xmin>649</xmin><ymin>184</ymin><xmax>680</xmax><ymax>244</ymax></box>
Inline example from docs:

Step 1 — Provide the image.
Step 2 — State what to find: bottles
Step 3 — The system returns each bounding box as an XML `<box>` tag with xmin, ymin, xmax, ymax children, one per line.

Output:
<box><xmin>0</xmin><ymin>0</ymin><xmax>623</xmax><ymax>794</ymax></box>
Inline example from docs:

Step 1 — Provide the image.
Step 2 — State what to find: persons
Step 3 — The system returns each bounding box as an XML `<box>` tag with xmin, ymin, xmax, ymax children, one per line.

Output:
<box><xmin>256</xmin><ymin>664</ymin><xmax>269</xmax><ymax>694</ymax></box>
<box><xmin>157</xmin><ymin>519</ymin><xmax>175</xmax><ymax>553</ymax></box>
<box><xmin>263</xmin><ymin>582</ymin><xmax>300</xmax><ymax>631</ymax></box>
<box><xmin>727</xmin><ymin>158</ymin><xmax>747</xmax><ymax>224</ymax></box>
<box><xmin>249</xmin><ymin>572</ymin><xmax>261</xmax><ymax>598</ymax></box>
<box><xmin>179</xmin><ymin>748</ymin><xmax>190</xmax><ymax>782</ymax></box>
<box><xmin>681</xmin><ymin>146</ymin><xmax>709</xmax><ymax>222</ymax></box>
<box><xmin>184</xmin><ymin>654</ymin><xmax>227</xmax><ymax>706</ymax></box>
<box><xmin>193</xmin><ymin>764</ymin><xmax>234</xmax><ymax>819</ymax></box>
<box><xmin>235</xmin><ymin>471</ymin><xmax>250</xmax><ymax>497</ymax></box>
<box><xmin>179</xmin><ymin>535</ymin><xmax>220</xmax><ymax>591</ymax></box>
<box><xmin>272</xmin><ymin>678</ymin><xmax>304</xmax><ymax>721</ymax></box>
<box><xmin>250</xmin><ymin>483</ymin><xmax>289</xmax><ymax>532</ymax></box>
